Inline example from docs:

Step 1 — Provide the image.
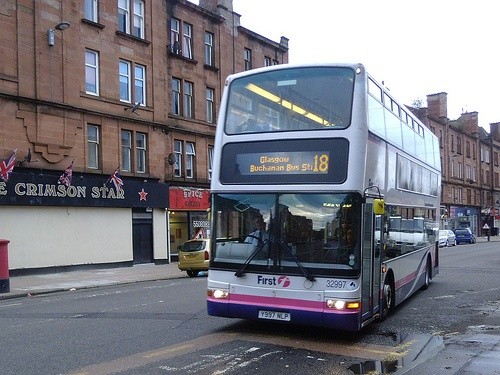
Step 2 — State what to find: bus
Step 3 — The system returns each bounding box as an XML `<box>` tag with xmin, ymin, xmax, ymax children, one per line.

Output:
<box><xmin>207</xmin><ymin>60</ymin><xmax>441</xmax><ymax>333</ymax></box>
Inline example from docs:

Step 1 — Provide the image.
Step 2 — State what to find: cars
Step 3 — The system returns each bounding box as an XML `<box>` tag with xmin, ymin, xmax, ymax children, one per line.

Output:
<box><xmin>376</xmin><ymin>217</ymin><xmax>429</xmax><ymax>247</ymax></box>
<box><xmin>438</xmin><ymin>229</ymin><xmax>453</xmax><ymax>246</ymax></box>
<box><xmin>453</xmin><ymin>227</ymin><xmax>476</xmax><ymax>245</ymax></box>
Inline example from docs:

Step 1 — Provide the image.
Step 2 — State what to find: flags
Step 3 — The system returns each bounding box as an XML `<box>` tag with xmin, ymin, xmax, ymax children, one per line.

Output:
<box><xmin>0</xmin><ymin>147</ymin><xmax>19</xmax><ymax>183</ymax></box>
<box><xmin>108</xmin><ymin>166</ymin><xmax>126</xmax><ymax>196</ymax></box>
<box><xmin>57</xmin><ymin>158</ymin><xmax>76</xmax><ymax>187</ymax></box>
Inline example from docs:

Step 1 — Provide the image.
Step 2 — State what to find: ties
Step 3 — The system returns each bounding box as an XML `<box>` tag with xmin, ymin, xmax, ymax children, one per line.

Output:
<box><xmin>258</xmin><ymin>232</ymin><xmax>263</xmax><ymax>247</ymax></box>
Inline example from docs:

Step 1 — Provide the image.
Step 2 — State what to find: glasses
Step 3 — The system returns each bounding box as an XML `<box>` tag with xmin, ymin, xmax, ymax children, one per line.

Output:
<box><xmin>341</xmin><ymin>221</ymin><xmax>348</xmax><ymax>224</ymax></box>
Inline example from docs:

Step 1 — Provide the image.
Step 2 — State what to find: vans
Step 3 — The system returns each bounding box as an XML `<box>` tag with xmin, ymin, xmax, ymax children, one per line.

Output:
<box><xmin>178</xmin><ymin>238</ymin><xmax>210</xmax><ymax>277</ymax></box>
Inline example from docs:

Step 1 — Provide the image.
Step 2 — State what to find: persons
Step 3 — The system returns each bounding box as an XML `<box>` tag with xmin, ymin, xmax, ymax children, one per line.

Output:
<box><xmin>244</xmin><ymin>216</ymin><xmax>270</xmax><ymax>258</ymax></box>
<box><xmin>235</xmin><ymin>114</ymin><xmax>261</xmax><ymax>132</ymax></box>
